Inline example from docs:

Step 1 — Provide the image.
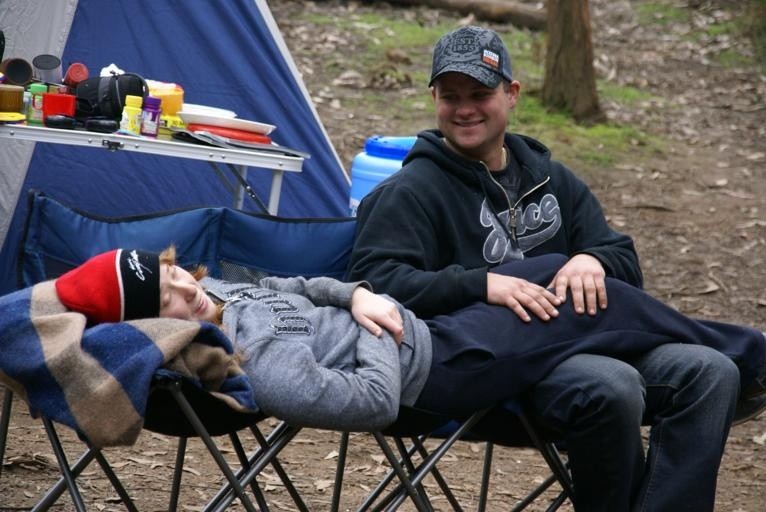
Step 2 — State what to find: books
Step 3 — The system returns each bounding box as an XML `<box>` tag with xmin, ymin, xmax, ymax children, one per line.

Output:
<box><xmin>170</xmin><ymin>124</ymin><xmax>313</xmax><ymax>160</ymax></box>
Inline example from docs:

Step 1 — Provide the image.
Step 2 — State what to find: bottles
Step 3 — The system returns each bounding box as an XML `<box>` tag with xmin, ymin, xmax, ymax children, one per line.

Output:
<box><xmin>120</xmin><ymin>94</ymin><xmax>161</xmax><ymax>137</ymax></box>
<box><xmin>28</xmin><ymin>84</ymin><xmax>48</xmax><ymax>126</ymax></box>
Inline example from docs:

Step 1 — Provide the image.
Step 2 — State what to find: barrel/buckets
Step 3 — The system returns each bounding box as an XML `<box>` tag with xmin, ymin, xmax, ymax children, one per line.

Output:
<box><xmin>345</xmin><ymin>134</ymin><xmax>417</xmax><ymax>217</ymax></box>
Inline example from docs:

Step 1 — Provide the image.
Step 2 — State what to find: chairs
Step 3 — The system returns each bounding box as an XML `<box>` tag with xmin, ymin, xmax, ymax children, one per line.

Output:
<box><xmin>0</xmin><ymin>190</ymin><xmax>357</xmax><ymax>512</ymax></box>
<box><xmin>356</xmin><ymin>397</ymin><xmax>578</xmax><ymax>511</ymax></box>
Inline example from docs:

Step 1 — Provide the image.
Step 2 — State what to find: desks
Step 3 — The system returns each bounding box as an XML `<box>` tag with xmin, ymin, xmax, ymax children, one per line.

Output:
<box><xmin>1</xmin><ymin>119</ymin><xmax>304</xmax><ymax>218</ymax></box>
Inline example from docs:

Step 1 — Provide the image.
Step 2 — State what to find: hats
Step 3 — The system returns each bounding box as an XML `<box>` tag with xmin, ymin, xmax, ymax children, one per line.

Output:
<box><xmin>53</xmin><ymin>248</ymin><xmax>161</xmax><ymax>323</ymax></box>
<box><xmin>426</xmin><ymin>24</ymin><xmax>513</xmax><ymax>91</ymax></box>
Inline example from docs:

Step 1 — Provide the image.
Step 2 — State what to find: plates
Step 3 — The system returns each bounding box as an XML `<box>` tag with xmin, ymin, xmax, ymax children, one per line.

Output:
<box><xmin>177</xmin><ymin>103</ymin><xmax>277</xmax><ymax>137</ymax></box>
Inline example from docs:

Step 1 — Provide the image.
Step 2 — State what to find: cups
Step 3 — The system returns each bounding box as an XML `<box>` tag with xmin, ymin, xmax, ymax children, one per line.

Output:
<box><xmin>32</xmin><ymin>93</ymin><xmax>76</xmax><ymax>125</ymax></box>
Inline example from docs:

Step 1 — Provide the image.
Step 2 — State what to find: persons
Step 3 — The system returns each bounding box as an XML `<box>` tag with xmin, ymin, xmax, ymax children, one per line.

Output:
<box><xmin>54</xmin><ymin>249</ymin><xmax>766</xmax><ymax>433</ymax></box>
<box><xmin>343</xmin><ymin>24</ymin><xmax>742</xmax><ymax>511</ymax></box>
